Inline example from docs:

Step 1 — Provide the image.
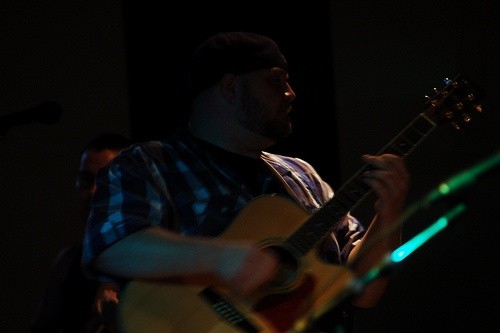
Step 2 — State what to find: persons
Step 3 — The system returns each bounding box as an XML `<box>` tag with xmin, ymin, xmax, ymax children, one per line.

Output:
<box><xmin>33</xmin><ymin>131</ymin><xmax>130</xmax><ymax>333</ymax></box>
<box><xmin>82</xmin><ymin>33</ymin><xmax>409</xmax><ymax>333</ymax></box>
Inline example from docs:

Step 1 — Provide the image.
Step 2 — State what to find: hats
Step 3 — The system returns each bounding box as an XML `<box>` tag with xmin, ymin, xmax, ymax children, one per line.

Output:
<box><xmin>179</xmin><ymin>33</ymin><xmax>287</xmax><ymax>101</ymax></box>
